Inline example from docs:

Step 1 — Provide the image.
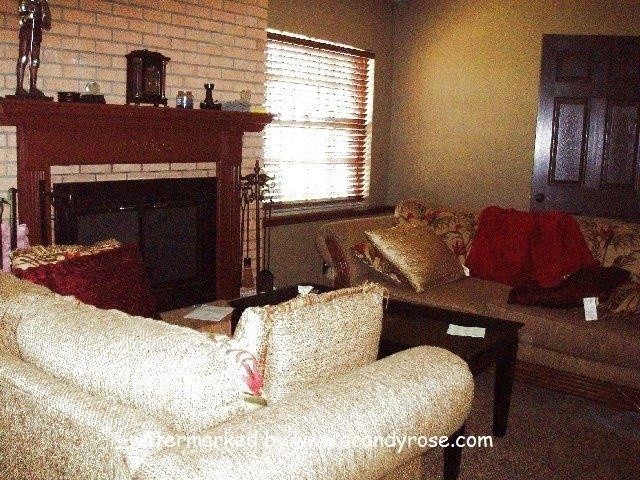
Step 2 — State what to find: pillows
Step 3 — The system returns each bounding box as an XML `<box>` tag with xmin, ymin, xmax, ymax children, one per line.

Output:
<box><xmin>232</xmin><ymin>288</ymin><xmax>386</xmax><ymax>405</ymax></box>
<box><xmin>10</xmin><ymin>241</ymin><xmax>163</xmax><ymax>335</ymax></box>
<box><xmin>364</xmin><ymin>218</ymin><xmax>463</xmax><ymax>296</ymax></box>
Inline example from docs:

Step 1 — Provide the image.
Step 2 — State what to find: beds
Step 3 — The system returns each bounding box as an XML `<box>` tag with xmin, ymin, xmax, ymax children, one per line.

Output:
<box><xmin>236</xmin><ymin>277</ymin><xmax>523</xmax><ymax>480</ymax></box>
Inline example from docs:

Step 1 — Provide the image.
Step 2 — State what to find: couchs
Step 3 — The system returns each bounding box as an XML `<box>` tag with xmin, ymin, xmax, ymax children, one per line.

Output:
<box><xmin>1</xmin><ymin>245</ymin><xmax>480</xmax><ymax>479</ymax></box>
<box><xmin>318</xmin><ymin>206</ymin><xmax>640</xmax><ymax>411</ymax></box>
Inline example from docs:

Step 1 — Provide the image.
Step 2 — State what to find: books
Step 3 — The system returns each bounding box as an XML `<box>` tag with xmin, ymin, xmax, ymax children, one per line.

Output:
<box><xmin>183</xmin><ymin>304</ymin><xmax>235</xmax><ymax>323</ymax></box>
<box><xmin>446</xmin><ymin>323</ymin><xmax>486</xmax><ymax>339</ymax></box>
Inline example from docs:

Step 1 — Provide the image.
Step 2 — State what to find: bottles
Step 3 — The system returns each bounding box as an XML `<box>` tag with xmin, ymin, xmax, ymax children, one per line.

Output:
<box><xmin>177</xmin><ymin>91</ymin><xmax>193</xmax><ymax>107</ymax></box>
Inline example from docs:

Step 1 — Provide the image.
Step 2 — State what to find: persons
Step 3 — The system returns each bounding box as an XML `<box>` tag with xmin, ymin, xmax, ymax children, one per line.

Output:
<box><xmin>16</xmin><ymin>0</ymin><xmax>52</xmax><ymax>97</ymax></box>
